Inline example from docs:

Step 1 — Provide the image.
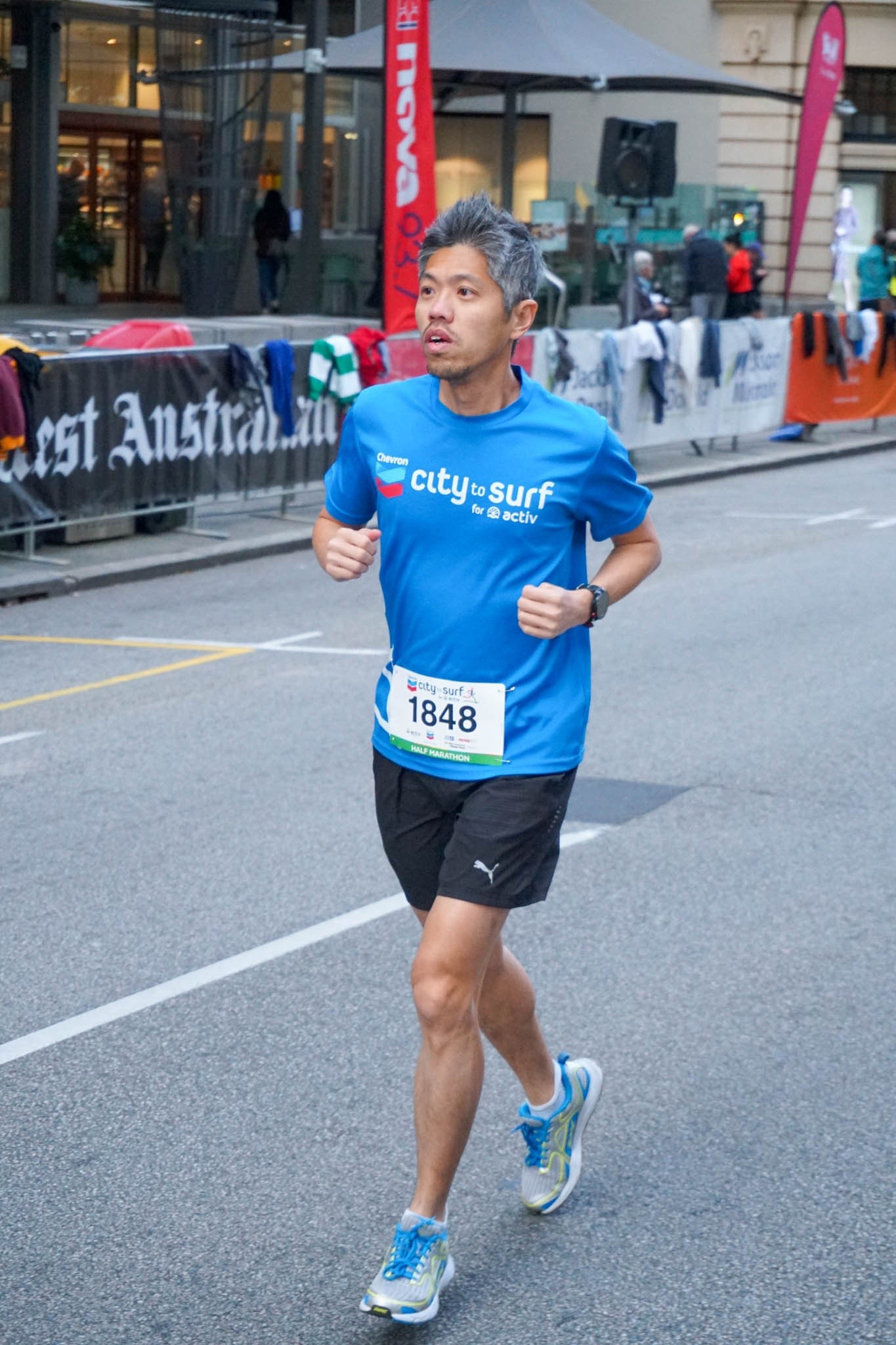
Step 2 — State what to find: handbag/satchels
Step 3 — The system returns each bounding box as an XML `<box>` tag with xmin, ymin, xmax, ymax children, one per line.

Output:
<box><xmin>265</xmin><ymin>237</ymin><xmax>292</xmax><ymax>260</ymax></box>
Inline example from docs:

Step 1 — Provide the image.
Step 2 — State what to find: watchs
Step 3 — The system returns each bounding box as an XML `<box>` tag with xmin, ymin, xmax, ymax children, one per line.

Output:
<box><xmin>575</xmin><ymin>584</ymin><xmax>611</xmax><ymax>631</ymax></box>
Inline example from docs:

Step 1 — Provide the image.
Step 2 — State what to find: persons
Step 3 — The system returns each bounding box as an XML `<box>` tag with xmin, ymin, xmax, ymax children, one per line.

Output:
<box><xmin>314</xmin><ymin>207</ymin><xmax>663</xmax><ymax>1321</ymax></box>
<box><xmin>618</xmin><ymin>220</ymin><xmax>770</xmax><ymax>328</ymax></box>
<box><xmin>53</xmin><ymin>159</ymin><xmax>84</xmax><ymax>298</ymax></box>
<box><xmin>138</xmin><ymin>170</ymin><xmax>171</xmax><ymax>295</ymax></box>
<box><xmin>856</xmin><ymin>227</ymin><xmax>896</xmax><ymax>316</ymax></box>
<box><xmin>253</xmin><ymin>188</ymin><xmax>292</xmax><ymax>316</ymax></box>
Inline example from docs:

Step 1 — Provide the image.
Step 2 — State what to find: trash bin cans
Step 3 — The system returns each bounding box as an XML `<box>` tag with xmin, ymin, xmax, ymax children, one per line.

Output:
<box><xmin>322</xmin><ymin>251</ymin><xmax>367</xmax><ymax>317</ymax></box>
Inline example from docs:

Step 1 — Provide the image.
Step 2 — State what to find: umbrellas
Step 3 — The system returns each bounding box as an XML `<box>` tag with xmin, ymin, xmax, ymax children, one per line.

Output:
<box><xmin>168</xmin><ymin>0</ymin><xmax>850</xmax><ymax>212</ymax></box>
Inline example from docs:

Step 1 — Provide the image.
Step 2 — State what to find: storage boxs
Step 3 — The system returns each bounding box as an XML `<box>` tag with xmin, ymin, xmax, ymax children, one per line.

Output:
<box><xmin>45</xmin><ymin>506</ymin><xmax>133</xmax><ymax>545</ymax></box>
<box><xmin>133</xmin><ymin>499</ymin><xmax>188</xmax><ymax>536</ymax></box>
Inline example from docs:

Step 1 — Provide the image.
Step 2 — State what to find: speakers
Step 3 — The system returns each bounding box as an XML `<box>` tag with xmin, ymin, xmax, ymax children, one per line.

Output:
<box><xmin>597</xmin><ymin>116</ymin><xmax>675</xmax><ymax>198</ymax></box>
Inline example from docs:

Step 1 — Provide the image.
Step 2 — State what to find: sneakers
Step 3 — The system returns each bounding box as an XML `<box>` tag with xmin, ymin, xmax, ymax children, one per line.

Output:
<box><xmin>359</xmin><ymin>1216</ymin><xmax>455</xmax><ymax>1326</ymax></box>
<box><xmin>509</xmin><ymin>1050</ymin><xmax>604</xmax><ymax>1215</ymax></box>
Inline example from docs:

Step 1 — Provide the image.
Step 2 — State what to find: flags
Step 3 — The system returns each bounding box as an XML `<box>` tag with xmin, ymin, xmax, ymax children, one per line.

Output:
<box><xmin>378</xmin><ymin>0</ymin><xmax>438</xmax><ymax>333</ymax></box>
<box><xmin>782</xmin><ymin>1</ymin><xmax>845</xmax><ymax>314</ymax></box>
